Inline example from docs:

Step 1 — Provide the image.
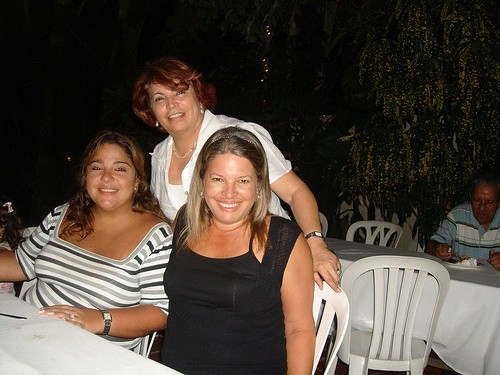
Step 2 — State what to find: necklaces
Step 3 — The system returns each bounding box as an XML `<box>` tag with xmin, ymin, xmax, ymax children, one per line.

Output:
<box><xmin>173</xmin><ymin>140</ymin><xmax>197</xmax><ymax>159</ymax></box>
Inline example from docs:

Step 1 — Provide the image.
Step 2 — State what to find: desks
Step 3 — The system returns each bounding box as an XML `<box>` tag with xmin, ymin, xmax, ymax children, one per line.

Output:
<box><xmin>0</xmin><ymin>290</ymin><xmax>187</xmax><ymax>375</ymax></box>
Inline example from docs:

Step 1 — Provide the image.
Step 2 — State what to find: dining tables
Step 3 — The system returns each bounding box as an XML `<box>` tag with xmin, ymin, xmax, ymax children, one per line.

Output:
<box><xmin>316</xmin><ymin>238</ymin><xmax>500</xmax><ymax>375</ymax></box>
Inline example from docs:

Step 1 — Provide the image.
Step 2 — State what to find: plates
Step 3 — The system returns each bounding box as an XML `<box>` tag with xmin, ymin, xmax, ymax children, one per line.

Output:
<box><xmin>443</xmin><ymin>261</ymin><xmax>485</xmax><ymax>269</ymax></box>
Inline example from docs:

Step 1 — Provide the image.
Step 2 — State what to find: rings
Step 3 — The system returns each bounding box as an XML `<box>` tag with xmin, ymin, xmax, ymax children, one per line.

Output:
<box><xmin>68</xmin><ymin>314</ymin><xmax>75</xmax><ymax>320</ymax></box>
<box><xmin>337</xmin><ymin>269</ymin><xmax>341</xmax><ymax>274</ymax></box>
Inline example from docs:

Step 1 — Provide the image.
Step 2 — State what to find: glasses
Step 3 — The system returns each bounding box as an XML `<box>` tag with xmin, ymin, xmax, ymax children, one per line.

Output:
<box><xmin>473</xmin><ymin>198</ymin><xmax>499</xmax><ymax>207</ymax></box>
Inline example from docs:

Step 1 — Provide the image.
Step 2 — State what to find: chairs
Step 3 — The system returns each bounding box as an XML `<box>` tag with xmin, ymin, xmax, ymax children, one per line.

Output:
<box><xmin>325</xmin><ymin>255</ymin><xmax>451</xmax><ymax>375</ymax></box>
<box><xmin>346</xmin><ymin>220</ymin><xmax>403</xmax><ymax>248</ymax></box>
<box><xmin>312</xmin><ymin>281</ymin><xmax>350</xmax><ymax>375</ymax></box>
<box><xmin>285</xmin><ymin>210</ymin><xmax>328</xmax><ymax>238</ymax></box>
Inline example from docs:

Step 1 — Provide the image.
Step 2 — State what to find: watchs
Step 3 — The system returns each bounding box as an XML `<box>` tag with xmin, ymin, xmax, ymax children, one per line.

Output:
<box><xmin>99</xmin><ymin>310</ymin><xmax>112</xmax><ymax>335</ymax></box>
<box><xmin>304</xmin><ymin>231</ymin><xmax>324</xmax><ymax>240</ymax></box>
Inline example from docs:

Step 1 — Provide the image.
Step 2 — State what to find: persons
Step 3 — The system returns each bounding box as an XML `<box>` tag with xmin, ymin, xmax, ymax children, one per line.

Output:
<box><xmin>132</xmin><ymin>58</ymin><xmax>342</xmax><ymax>294</ymax></box>
<box><xmin>426</xmin><ymin>171</ymin><xmax>500</xmax><ymax>271</ymax></box>
<box><xmin>0</xmin><ymin>202</ymin><xmax>23</xmax><ymax>296</ymax></box>
<box><xmin>0</xmin><ymin>132</ymin><xmax>174</xmax><ymax>357</ymax></box>
<box><xmin>160</xmin><ymin>126</ymin><xmax>316</xmax><ymax>375</ymax></box>
